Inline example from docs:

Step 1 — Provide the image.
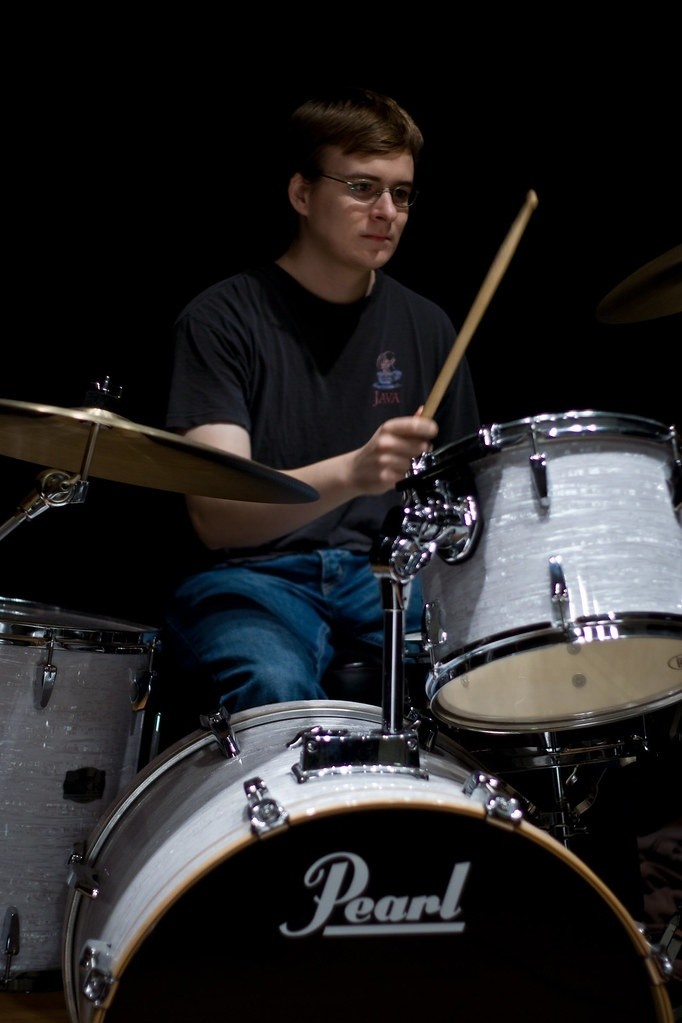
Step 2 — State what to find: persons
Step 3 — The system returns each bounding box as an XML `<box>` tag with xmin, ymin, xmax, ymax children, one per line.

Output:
<box><xmin>160</xmin><ymin>90</ymin><xmax>481</xmax><ymax>743</ymax></box>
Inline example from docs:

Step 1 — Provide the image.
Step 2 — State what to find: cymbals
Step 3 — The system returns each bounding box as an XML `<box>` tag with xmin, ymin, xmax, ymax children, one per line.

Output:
<box><xmin>0</xmin><ymin>397</ymin><xmax>322</xmax><ymax>505</ymax></box>
<box><xmin>596</xmin><ymin>242</ymin><xmax>681</xmax><ymax>326</ymax></box>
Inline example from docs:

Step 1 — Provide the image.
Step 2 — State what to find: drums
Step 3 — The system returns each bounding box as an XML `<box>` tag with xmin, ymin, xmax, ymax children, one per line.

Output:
<box><xmin>471</xmin><ymin>740</ymin><xmax>646</xmax><ymax>921</ymax></box>
<box><xmin>400</xmin><ymin>408</ymin><xmax>682</xmax><ymax>733</ymax></box>
<box><xmin>60</xmin><ymin>701</ymin><xmax>675</xmax><ymax>1023</ymax></box>
<box><xmin>0</xmin><ymin>597</ymin><xmax>159</xmax><ymax>994</ymax></box>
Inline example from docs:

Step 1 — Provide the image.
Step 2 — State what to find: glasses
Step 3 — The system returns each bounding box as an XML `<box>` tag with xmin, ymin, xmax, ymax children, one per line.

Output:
<box><xmin>308</xmin><ymin>171</ymin><xmax>420</xmax><ymax>208</ymax></box>
